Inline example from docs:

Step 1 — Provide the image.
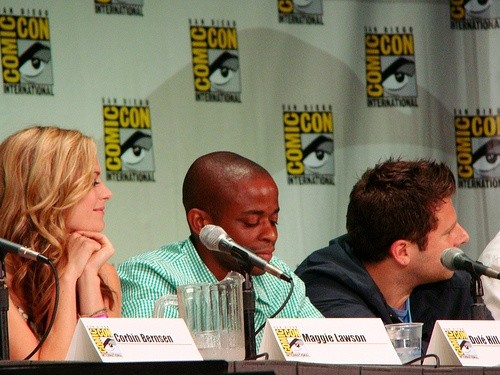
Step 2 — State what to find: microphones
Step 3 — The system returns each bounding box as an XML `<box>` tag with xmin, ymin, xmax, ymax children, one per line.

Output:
<box><xmin>439</xmin><ymin>247</ymin><xmax>500</xmax><ymax>279</ymax></box>
<box><xmin>198</xmin><ymin>223</ymin><xmax>294</xmax><ymax>282</ymax></box>
<box><xmin>0</xmin><ymin>237</ymin><xmax>49</xmax><ymax>264</ymax></box>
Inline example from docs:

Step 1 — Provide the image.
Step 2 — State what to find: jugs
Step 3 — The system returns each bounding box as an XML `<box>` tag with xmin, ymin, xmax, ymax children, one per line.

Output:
<box><xmin>153</xmin><ymin>270</ymin><xmax>247</xmax><ymax>361</ymax></box>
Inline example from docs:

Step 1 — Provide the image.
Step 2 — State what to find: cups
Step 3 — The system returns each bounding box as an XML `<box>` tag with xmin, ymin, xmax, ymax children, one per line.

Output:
<box><xmin>386</xmin><ymin>322</ymin><xmax>424</xmax><ymax>365</ymax></box>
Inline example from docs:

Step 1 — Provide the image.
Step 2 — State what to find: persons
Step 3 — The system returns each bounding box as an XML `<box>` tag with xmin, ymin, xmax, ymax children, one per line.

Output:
<box><xmin>476</xmin><ymin>231</ymin><xmax>500</xmax><ymax>320</ymax></box>
<box><xmin>116</xmin><ymin>151</ymin><xmax>326</xmax><ymax>354</ymax></box>
<box><xmin>0</xmin><ymin>127</ymin><xmax>122</xmax><ymax>362</ymax></box>
<box><xmin>294</xmin><ymin>162</ymin><xmax>472</xmax><ymax>323</ymax></box>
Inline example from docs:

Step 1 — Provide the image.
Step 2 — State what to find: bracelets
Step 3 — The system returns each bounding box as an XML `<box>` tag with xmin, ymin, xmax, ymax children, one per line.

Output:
<box><xmin>94</xmin><ymin>314</ymin><xmax>108</xmax><ymax>318</ymax></box>
<box><xmin>82</xmin><ymin>308</ymin><xmax>107</xmax><ymax>318</ymax></box>
